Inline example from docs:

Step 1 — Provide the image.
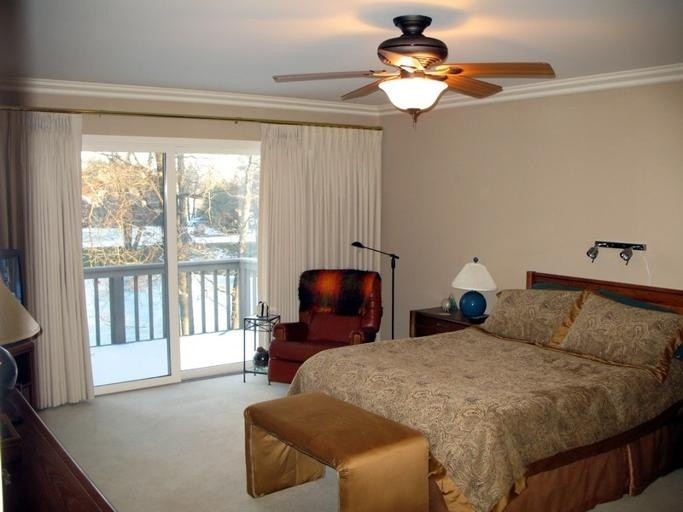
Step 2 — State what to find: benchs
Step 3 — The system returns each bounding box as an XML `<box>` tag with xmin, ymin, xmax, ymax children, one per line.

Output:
<box><xmin>242</xmin><ymin>390</ymin><xmax>431</xmax><ymax>512</ymax></box>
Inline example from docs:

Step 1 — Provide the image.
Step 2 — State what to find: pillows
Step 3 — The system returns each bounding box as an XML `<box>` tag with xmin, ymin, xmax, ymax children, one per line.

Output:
<box><xmin>471</xmin><ymin>287</ymin><xmax>682</xmax><ymax>384</ymax></box>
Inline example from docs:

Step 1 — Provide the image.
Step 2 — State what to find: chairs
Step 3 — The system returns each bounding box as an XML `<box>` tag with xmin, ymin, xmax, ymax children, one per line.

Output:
<box><xmin>267</xmin><ymin>269</ymin><xmax>383</xmax><ymax>387</ymax></box>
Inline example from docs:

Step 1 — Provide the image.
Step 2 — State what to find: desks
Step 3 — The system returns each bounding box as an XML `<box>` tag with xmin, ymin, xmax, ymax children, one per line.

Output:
<box><xmin>243</xmin><ymin>314</ymin><xmax>281</xmax><ymax>386</ymax></box>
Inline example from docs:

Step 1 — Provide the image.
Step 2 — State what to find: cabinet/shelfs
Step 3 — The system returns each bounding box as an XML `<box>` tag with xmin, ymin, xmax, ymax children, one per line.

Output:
<box><xmin>0</xmin><ymin>338</ymin><xmax>119</xmax><ymax>511</ymax></box>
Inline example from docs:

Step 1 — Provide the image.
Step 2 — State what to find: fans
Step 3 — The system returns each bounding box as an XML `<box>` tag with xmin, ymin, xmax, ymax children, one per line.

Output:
<box><xmin>271</xmin><ymin>15</ymin><xmax>557</xmax><ymax>101</ymax></box>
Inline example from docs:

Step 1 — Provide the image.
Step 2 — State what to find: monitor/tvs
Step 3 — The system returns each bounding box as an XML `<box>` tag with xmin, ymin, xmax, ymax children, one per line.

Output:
<box><xmin>0</xmin><ymin>247</ymin><xmax>25</xmax><ymax>306</ymax></box>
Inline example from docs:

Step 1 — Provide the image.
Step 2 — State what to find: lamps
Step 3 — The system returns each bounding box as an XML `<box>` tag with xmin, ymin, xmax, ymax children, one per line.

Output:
<box><xmin>586</xmin><ymin>241</ymin><xmax>644</xmax><ymax>266</ymax></box>
<box><xmin>2</xmin><ymin>275</ymin><xmax>42</xmax><ymax>425</ymax></box>
<box><xmin>450</xmin><ymin>258</ymin><xmax>498</xmax><ymax>321</ymax></box>
<box><xmin>378</xmin><ymin>72</ymin><xmax>449</xmax><ymax>124</ymax></box>
<box><xmin>351</xmin><ymin>241</ymin><xmax>400</xmax><ymax>339</ymax></box>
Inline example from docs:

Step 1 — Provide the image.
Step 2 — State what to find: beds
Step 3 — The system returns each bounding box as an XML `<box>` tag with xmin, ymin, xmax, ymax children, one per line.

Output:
<box><xmin>285</xmin><ymin>270</ymin><xmax>682</xmax><ymax>512</ymax></box>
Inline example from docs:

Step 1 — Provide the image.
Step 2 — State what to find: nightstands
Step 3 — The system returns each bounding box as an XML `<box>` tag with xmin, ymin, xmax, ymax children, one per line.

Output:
<box><xmin>409</xmin><ymin>306</ymin><xmax>491</xmax><ymax>338</ymax></box>
